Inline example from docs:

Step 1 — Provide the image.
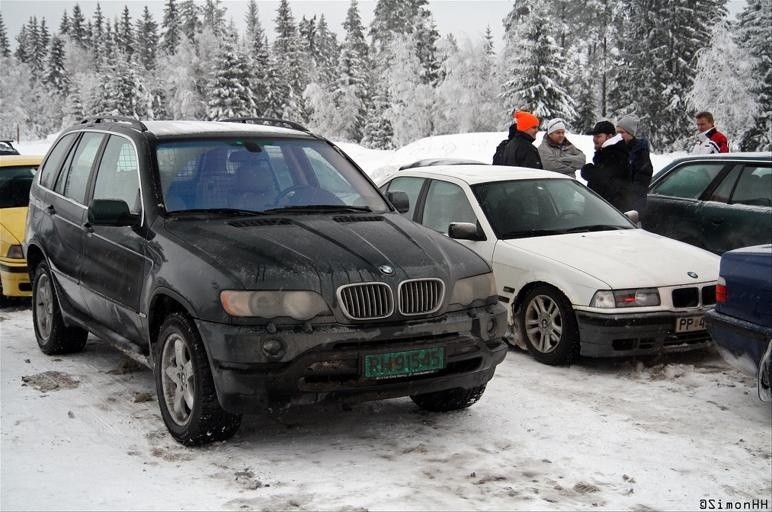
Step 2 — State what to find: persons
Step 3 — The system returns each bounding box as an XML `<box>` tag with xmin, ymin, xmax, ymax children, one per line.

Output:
<box><xmin>615</xmin><ymin>114</ymin><xmax>652</xmax><ymax>214</ymax></box>
<box><xmin>537</xmin><ymin>117</ymin><xmax>587</xmax><ymax>181</ymax></box>
<box><xmin>580</xmin><ymin>121</ymin><xmax>633</xmax><ymax>214</ymax></box>
<box><xmin>501</xmin><ymin>110</ymin><xmax>543</xmax><ymax>170</ymax></box>
<box><xmin>493</xmin><ymin>124</ymin><xmax>516</xmax><ymax>164</ymax></box>
<box><xmin>693</xmin><ymin>111</ymin><xmax>728</xmax><ymax>154</ymax></box>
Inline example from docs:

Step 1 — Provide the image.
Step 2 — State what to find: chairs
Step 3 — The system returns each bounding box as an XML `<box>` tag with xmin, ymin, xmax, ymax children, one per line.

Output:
<box><xmin>498</xmin><ymin>198</ymin><xmax>542</xmax><ymax>233</ymax></box>
<box><xmin>197</xmin><ymin>163</ymin><xmax>234</xmax><ymax>210</ymax></box>
<box><xmin>231</xmin><ymin>168</ymin><xmax>288</xmax><ymax>212</ymax></box>
<box><xmin>117</xmin><ymin>172</ymin><xmax>186</xmax><ymax>215</ymax></box>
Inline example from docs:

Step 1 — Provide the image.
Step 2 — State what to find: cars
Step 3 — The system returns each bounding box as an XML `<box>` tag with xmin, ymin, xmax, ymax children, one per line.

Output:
<box><xmin>0</xmin><ymin>140</ymin><xmax>43</xmax><ymax>306</ymax></box>
<box><xmin>380</xmin><ymin>155</ymin><xmax>771</xmax><ymax>401</ymax></box>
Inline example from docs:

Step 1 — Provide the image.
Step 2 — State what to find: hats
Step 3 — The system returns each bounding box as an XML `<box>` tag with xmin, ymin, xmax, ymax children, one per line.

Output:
<box><xmin>548</xmin><ymin>120</ymin><xmax>565</xmax><ymax>134</ymax></box>
<box><xmin>617</xmin><ymin>117</ymin><xmax>637</xmax><ymax>137</ymax></box>
<box><xmin>587</xmin><ymin>121</ymin><xmax>615</xmax><ymax>134</ymax></box>
<box><xmin>514</xmin><ymin>112</ymin><xmax>539</xmax><ymax>132</ymax></box>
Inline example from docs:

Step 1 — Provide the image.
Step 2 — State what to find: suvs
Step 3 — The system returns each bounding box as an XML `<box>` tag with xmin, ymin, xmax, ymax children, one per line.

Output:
<box><xmin>24</xmin><ymin>116</ymin><xmax>507</xmax><ymax>445</ymax></box>
